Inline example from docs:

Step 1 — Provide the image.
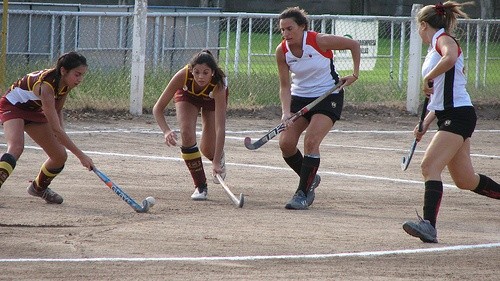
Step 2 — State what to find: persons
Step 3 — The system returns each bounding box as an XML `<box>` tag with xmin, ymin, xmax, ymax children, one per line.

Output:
<box><xmin>0</xmin><ymin>50</ymin><xmax>94</xmax><ymax>204</ymax></box>
<box><xmin>153</xmin><ymin>48</ymin><xmax>229</xmax><ymax>199</ymax></box>
<box><xmin>275</xmin><ymin>7</ymin><xmax>360</xmax><ymax>209</ymax></box>
<box><xmin>400</xmin><ymin>0</ymin><xmax>500</xmax><ymax>244</ymax></box>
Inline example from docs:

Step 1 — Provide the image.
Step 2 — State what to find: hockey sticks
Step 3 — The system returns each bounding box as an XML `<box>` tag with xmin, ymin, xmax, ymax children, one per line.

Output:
<box><xmin>90</xmin><ymin>166</ymin><xmax>149</xmax><ymax>213</ymax></box>
<box><xmin>244</xmin><ymin>80</ymin><xmax>346</xmax><ymax>150</ymax></box>
<box><xmin>401</xmin><ymin>78</ymin><xmax>434</xmax><ymax>171</ymax></box>
<box><xmin>216</xmin><ymin>173</ymin><xmax>244</xmax><ymax>208</ymax></box>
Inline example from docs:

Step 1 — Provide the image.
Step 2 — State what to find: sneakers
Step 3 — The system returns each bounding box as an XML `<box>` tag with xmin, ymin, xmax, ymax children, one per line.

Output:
<box><xmin>27</xmin><ymin>179</ymin><xmax>64</xmax><ymax>205</ymax></box>
<box><xmin>284</xmin><ymin>190</ymin><xmax>309</xmax><ymax>210</ymax></box>
<box><xmin>401</xmin><ymin>210</ymin><xmax>438</xmax><ymax>242</ymax></box>
<box><xmin>212</xmin><ymin>151</ymin><xmax>227</xmax><ymax>184</ymax></box>
<box><xmin>192</xmin><ymin>185</ymin><xmax>209</xmax><ymax>200</ymax></box>
<box><xmin>306</xmin><ymin>174</ymin><xmax>321</xmax><ymax>207</ymax></box>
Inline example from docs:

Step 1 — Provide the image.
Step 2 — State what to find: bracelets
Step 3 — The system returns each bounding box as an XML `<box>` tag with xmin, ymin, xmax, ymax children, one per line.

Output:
<box><xmin>353</xmin><ymin>74</ymin><xmax>358</xmax><ymax>79</ymax></box>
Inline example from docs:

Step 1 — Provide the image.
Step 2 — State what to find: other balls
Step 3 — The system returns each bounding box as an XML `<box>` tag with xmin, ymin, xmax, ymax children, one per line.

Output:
<box><xmin>146</xmin><ymin>196</ymin><xmax>155</xmax><ymax>207</ymax></box>
